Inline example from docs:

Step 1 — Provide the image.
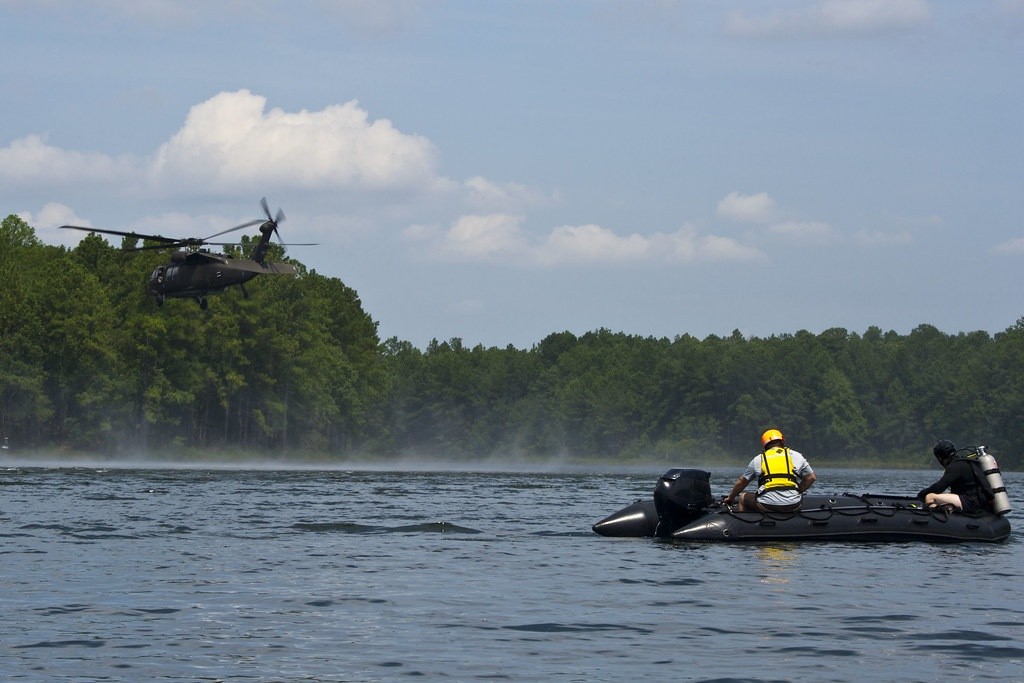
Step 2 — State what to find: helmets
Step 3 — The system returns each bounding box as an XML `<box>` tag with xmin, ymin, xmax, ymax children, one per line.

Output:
<box><xmin>761</xmin><ymin>429</ymin><xmax>783</xmax><ymax>447</ymax></box>
<box><xmin>933</xmin><ymin>440</ymin><xmax>956</xmax><ymax>459</ymax></box>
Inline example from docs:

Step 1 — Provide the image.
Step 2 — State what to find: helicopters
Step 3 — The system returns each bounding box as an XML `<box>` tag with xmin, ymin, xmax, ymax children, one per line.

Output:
<box><xmin>58</xmin><ymin>195</ymin><xmax>322</xmax><ymax>313</ymax></box>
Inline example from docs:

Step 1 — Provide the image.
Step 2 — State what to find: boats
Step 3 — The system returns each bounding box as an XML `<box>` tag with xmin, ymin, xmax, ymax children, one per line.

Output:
<box><xmin>592</xmin><ymin>463</ymin><xmax>1013</xmax><ymax>544</ymax></box>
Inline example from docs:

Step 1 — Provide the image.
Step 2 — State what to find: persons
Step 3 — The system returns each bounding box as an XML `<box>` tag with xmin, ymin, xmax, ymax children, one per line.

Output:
<box><xmin>724</xmin><ymin>429</ymin><xmax>816</xmax><ymax>514</ymax></box>
<box><xmin>917</xmin><ymin>440</ymin><xmax>995</xmax><ymax>514</ymax></box>
